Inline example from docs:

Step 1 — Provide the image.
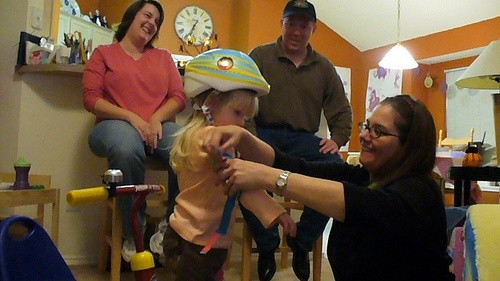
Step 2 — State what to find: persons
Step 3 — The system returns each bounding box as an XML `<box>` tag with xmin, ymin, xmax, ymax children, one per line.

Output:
<box><xmin>198</xmin><ymin>95</ymin><xmax>456</xmax><ymax>281</ymax></box>
<box><xmin>82</xmin><ymin>0</ymin><xmax>187</xmax><ymax>262</ymax></box>
<box><xmin>239</xmin><ymin>0</ymin><xmax>353</xmax><ymax>281</ymax></box>
<box><xmin>149</xmin><ymin>48</ymin><xmax>297</xmax><ymax>281</ymax></box>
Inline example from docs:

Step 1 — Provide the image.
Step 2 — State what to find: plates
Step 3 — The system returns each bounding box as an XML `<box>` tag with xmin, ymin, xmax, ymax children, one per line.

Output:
<box><xmin>61</xmin><ymin>0</ymin><xmax>80</xmax><ymax>17</ymax></box>
<box><xmin>0</xmin><ymin>183</ymin><xmax>14</xmax><ymax>190</ymax></box>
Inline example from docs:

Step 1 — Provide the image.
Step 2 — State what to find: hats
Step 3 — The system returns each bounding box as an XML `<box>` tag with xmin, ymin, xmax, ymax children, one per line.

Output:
<box><xmin>283</xmin><ymin>0</ymin><xmax>316</xmax><ymax>21</ymax></box>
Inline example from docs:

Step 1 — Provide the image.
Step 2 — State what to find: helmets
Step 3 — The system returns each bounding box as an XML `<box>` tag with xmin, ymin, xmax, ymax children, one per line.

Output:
<box><xmin>184</xmin><ymin>49</ymin><xmax>270</xmax><ymax>99</ymax></box>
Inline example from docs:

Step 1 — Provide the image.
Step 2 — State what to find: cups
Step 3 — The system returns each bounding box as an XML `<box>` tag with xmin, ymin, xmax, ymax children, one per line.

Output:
<box><xmin>451</xmin><ymin>150</ymin><xmax>465</xmax><ymax>167</ymax></box>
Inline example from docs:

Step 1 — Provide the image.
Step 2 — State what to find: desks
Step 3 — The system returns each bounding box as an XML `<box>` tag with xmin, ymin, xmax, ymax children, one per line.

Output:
<box><xmin>448</xmin><ymin>166</ymin><xmax>500</xmax><ymax>208</ymax></box>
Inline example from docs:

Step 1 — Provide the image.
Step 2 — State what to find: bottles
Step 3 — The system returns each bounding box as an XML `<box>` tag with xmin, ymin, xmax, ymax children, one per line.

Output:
<box><xmin>12</xmin><ymin>159</ymin><xmax>31</xmax><ymax>190</ymax></box>
<box><xmin>89</xmin><ymin>9</ymin><xmax>108</xmax><ymax>29</ymax></box>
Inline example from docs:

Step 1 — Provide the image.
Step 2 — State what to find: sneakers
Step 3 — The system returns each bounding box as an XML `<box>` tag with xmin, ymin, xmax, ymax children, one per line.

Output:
<box><xmin>122</xmin><ymin>239</ymin><xmax>136</xmax><ymax>262</ymax></box>
<box><xmin>150</xmin><ymin>218</ymin><xmax>169</xmax><ymax>259</ymax></box>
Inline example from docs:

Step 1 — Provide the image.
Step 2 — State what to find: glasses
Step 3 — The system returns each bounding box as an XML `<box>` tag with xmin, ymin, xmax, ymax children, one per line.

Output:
<box><xmin>358</xmin><ymin>121</ymin><xmax>401</xmax><ymax>139</ymax></box>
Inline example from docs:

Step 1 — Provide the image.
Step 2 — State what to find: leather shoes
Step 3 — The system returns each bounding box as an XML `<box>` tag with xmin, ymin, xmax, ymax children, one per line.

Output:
<box><xmin>258</xmin><ymin>240</ymin><xmax>280</xmax><ymax>281</ymax></box>
<box><xmin>286</xmin><ymin>233</ymin><xmax>310</xmax><ymax>281</ymax></box>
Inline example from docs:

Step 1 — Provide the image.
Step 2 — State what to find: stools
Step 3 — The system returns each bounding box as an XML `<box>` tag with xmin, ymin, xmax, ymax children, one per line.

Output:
<box><xmin>225</xmin><ymin>191</ymin><xmax>322</xmax><ymax>281</ymax></box>
<box><xmin>96</xmin><ymin>158</ymin><xmax>179</xmax><ymax>281</ymax></box>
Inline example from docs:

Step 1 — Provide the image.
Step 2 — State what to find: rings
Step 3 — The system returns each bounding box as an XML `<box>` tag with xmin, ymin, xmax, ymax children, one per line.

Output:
<box><xmin>226</xmin><ymin>180</ymin><xmax>233</xmax><ymax>184</ymax></box>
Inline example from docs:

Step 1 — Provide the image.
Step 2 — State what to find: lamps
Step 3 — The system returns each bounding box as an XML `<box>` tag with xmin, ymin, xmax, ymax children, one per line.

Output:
<box><xmin>377</xmin><ymin>0</ymin><xmax>418</xmax><ymax>70</ymax></box>
<box><xmin>455</xmin><ymin>40</ymin><xmax>500</xmax><ymax>167</ymax></box>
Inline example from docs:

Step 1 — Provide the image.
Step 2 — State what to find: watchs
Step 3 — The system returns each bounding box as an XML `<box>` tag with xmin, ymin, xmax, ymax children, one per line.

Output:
<box><xmin>276</xmin><ymin>171</ymin><xmax>291</xmax><ymax>197</ymax></box>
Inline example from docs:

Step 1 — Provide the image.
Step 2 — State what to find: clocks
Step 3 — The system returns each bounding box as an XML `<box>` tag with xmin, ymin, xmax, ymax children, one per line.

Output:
<box><xmin>175</xmin><ymin>5</ymin><xmax>215</xmax><ymax>46</ymax></box>
